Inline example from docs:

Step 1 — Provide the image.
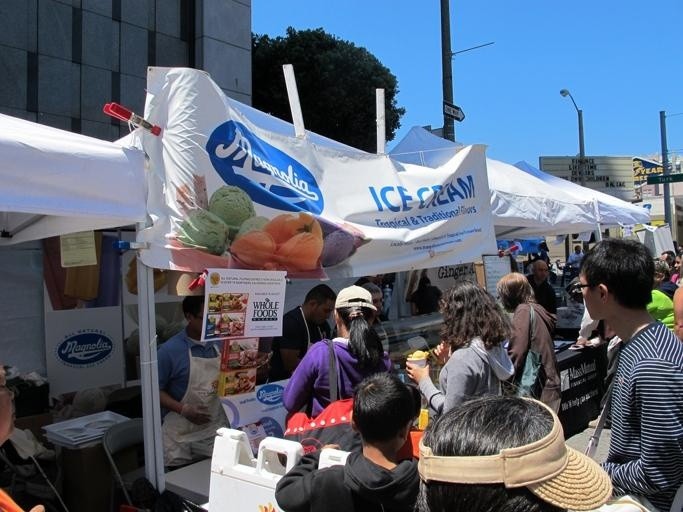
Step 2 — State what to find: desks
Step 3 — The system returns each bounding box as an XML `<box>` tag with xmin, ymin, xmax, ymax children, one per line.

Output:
<box><xmin>550</xmin><ymin>328</ymin><xmax>609</xmax><ymax>367</ymax></box>
<box><xmin>164</xmin><ymin>457</ymin><xmax>211</xmax><ymax>512</ymax></box>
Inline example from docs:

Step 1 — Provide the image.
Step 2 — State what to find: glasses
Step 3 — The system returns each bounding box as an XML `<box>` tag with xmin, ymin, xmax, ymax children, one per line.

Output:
<box><xmin>570</xmin><ymin>281</ymin><xmax>593</xmax><ymax>294</ymax></box>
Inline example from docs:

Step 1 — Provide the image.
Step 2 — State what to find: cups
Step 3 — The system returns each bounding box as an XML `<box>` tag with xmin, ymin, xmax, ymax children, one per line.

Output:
<box><xmin>407</xmin><ymin>358</ymin><xmax>426</xmax><ymax>380</ymax></box>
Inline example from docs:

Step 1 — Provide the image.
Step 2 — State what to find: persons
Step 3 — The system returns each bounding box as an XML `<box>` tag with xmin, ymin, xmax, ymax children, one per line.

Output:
<box><xmin>579</xmin><ymin>237</ymin><xmax>683</xmax><ymax>511</ymax></box>
<box><xmin>273</xmin><ymin>370</ymin><xmax>419</xmax><ymax>511</ymax></box>
<box><xmin>156</xmin><ymin>294</ymin><xmax>224</xmax><ymax>473</ymax></box>
<box><xmin>0</xmin><ymin>361</ymin><xmax>44</xmax><ymax>511</ymax></box>
<box><xmin>421</xmin><ymin>393</ymin><xmax>575</xmax><ymax>512</ymax></box>
<box><xmin>276</xmin><ymin>237</ymin><xmax>683</xmax><ymax>419</ymax></box>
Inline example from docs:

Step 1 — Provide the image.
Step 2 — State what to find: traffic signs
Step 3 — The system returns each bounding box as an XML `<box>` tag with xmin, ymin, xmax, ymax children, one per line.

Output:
<box><xmin>444</xmin><ymin>100</ymin><xmax>467</xmax><ymax>123</ymax></box>
<box><xmin>646</xmin><ymin>173</ymin><xmax>682</xmax><ymax>184</ymax></box>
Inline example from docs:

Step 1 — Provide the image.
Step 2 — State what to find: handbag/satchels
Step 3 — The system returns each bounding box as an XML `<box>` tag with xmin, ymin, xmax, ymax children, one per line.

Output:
<box><xmin>567</xmin><ymin>490</ymin><xmax>659</xmax><ymax>512</ymax></box>
<box><xmin>501</xmin><ymin>304</ymin><xmax>544</xmax><ymax>399</ymax></box>
<box><xmin>282</xmin><ymin>339</ymin><xmax>362</xmax><ymax>456</ymax></box>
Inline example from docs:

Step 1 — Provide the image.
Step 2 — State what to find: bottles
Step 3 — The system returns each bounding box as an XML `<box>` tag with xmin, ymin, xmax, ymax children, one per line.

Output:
<box><xmin>561</xmin><ymin>295</ymin><xmax>567</xmax><ymax>307</ymax></box>
<box><xmin>383</xmin><ymin>285</ymin><xmax>392</xmax><ymax>310</ymax></box>
<box><xmin>224</xmin><ymin>341</ymin><xmax>240</xmax><ymax>395</ymax></box>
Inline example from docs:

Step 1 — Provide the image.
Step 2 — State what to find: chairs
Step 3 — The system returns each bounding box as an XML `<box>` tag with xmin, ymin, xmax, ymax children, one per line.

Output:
<box><xmin>101</xmin><ymin>418</ymin><xmax>142</xmax><ymax>511</ymax></box>
<box><xmin>0</xmin><ymin>442</ymin><xmax>70</xmax><ymax>512</ymax></box>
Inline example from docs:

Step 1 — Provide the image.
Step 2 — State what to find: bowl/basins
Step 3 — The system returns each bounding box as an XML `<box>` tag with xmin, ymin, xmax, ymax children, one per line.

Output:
<box><xmin>168</xmin><ymin>240</ymin><xmax>229</xmax><ymax>272</ymax></box>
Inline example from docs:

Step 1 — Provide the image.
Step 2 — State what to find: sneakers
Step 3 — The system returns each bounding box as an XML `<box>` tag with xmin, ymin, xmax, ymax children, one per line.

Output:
<box><xmin>587</xmin><ymin>415</ymin><xmax>611</xmax><ymax>428</ymax></box>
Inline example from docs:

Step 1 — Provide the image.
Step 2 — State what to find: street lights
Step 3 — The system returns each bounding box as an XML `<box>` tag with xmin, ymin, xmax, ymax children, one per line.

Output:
<box><xmin>558</xmin><ymin>86</ymin><xmax>585</xmax><ymax>186</ymax></box>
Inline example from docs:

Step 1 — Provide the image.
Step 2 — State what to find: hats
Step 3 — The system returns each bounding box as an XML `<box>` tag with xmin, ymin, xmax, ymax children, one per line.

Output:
<box><xmin>417</xmin><ymin>398</ymin><xmax>613</xmax><ymax>512</ymax></box>
<box><xmin>334</xmin><ymin>285</ymin><xmax>377</xmax><ymax>311</ymax></box>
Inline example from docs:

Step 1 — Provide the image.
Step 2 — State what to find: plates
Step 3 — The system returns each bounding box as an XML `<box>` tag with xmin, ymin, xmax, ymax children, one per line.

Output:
<box><xmin>227</xmin><ymin>238</ymin><xmax>375</xmax><ymax>281</ymax></box>
<box><xmin>237</xmin><ymin>349</ymin><xmax>258</xmax><ymax>392</ymax></box>
